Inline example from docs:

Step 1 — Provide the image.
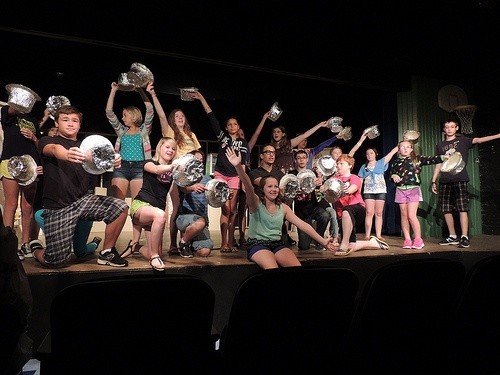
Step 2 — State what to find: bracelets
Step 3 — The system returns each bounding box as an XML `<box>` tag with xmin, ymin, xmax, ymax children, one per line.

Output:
<box><xmin>357</xmin><ymin>142</ymin><xmax>361</xmax><ymax>146</ymax></box>
<box><xmin>336</xmin><ymin>135</ymin><xmax>338</xmax><ymax>138</ymax></box>
<box><xmin>152</xmin><ymin>94</ymin><xmax>157</xmax><ymax>98</ymax></box>
<box><xmin>432</xmin><ymin>182</ymin><xmax>436</xmax><ymax>184</ymax></box>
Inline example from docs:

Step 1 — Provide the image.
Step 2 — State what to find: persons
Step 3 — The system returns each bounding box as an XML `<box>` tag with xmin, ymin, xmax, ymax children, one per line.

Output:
<box><xmin>31</xmin><ymin>124</ymin><xmax>60</xmax><ymax>241</ymax></box>
<box><xmin>29</xmin><ymin>105</ymin><xmax>128</xmax><ymax>267</ymax></box>
<box><xmin>0</xmin><ymin>100</ymin><xmax>49</xmax><ymax>250</ymax></box>
<box><xmin>358</xmin><ymin>145</ymin><xmax>399</xmax><ymax>242</ymax></box>
<box><xmin>0</xmin><ymin>105</ymin><xmax>41</xmax><ymax>261</ymax></box>
<box><xmin>173</xmin><ymin>150</ymin><xmax>215</xmax><ymax>257</ymax></box>
<box><xmin>225</xmin><ymin>146</ymin><xmax>337</xmax><ymax>269</ymax></box>
<box><xmin>292</xmin><ymin>133</ymin><xmax>342</xmax><ymax>170</ymax></box>
<box><xmin>271</xmin><ymin>121</ymin><xmax>328</xmax><ymax>175</ymax></box>
<box><xmin>146</xmin><ymin>81</ymin><xmax>202</xmax><ymax>255</ymax></box>
<box><xmin>106</xmin><ymin>81</ymin><xmax>153</xmax><ymax>255</ymax></box>
<box><xmin>119</xmin><ymin>137</ymin><xmax>178</xmax><ymax>272</ymax></box>
<box><xmin>389</xmin><ymin>140</ymin><xmax>449</xmax><ymax>250</ymax></box>
<box><xmin>288</xmin><ymin>150</ymin><xmax>331</xmax><ymax>251</ymax></box>
<box><xmin>431</xmin><ymin>118</ymin><xmax>500</xmax><ymax>247</ymax></box>
<box><xmin>330</xmin><ymin>133</ymin><xmax>367</xmax><ymax>160</ymax></box>
<box><xmin>249</xmin><ymin>144</ymin><xmax>297</xmax><ymax>247</ymax></box>
<box><xmin>34</xmin><ymin>165</ymin><xmax>102</xmax><ymax>257</ymax></box>
<box><xmin>320</xmin><ymin>154</ymin><xmax>390</xmax><ymax>255</ymax></box>
<box><xmin>190</xmin><ymin>91</ymin><xmax>250</xmax><ymax>253</ymax></box>
<box><xmin>311</xmin><ymin>161</ymin><xmax>339</xmax><ymax>247</ymax></box>
<box><xmin>233</xmin><ymin>111</ymin><xmax>271</xmax><ymax>247</ymax></box>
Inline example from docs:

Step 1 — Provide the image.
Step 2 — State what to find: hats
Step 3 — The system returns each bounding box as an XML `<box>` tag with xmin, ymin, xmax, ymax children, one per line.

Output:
<box><xmin>317</xmin><ymin>155</ymin><xmax>337</xmax><ymax>177</ymax></box>
<box><xmin>267</xmin><ymin>102</ymin><xmax>283</xmax><ymax>122</ymax></box>
<box><xmin>363</xmin><ymin>125</ymin><xmax>380</xmax><ymax>139</ymax></box>
<box><xmin>338</xmin><ymin>127</ymin><xmax>353</xmax><ymax>141</ymax></box>
<box><xmin>126</xmin><ymin>63</ymin><xmax>154</xmax><ymax>88</ymax></box>
<box><xmin>172</xmin><ymin>154</ymin><xmax>204</xmax><ymax>187</ymax></box>
<box><xmin>179</xmin><ymin>87</ymin><xmax>198</xmax><ymax>101</ymax></box>
<box><xmin>46</xmin><ymin>95</ymin><xmax>71</xmax><ymax>121</ymax></box>
<box><xmin>204</xmin><ymin>178</ymin><xmax>230</xmax><ymax>208</ymax></box>
<box><xmin>403</xmin><ymin>129</ymin><xmax>421</xmax><ymax>144</ymax></box>
<box><xmin>321</xmin><ymin>178</ymin><xmax>345</xmax><ymax>203</ymax></box>
<box><xmin>440</xmin><ymin>152</ymin><xmax>466</xmax><ymax>176</ymax></box>
<box><xmin>296</xmin><ymin>169</ymin><xmax>317</xmax><ymax>194</ymax></box>
<box><xmin>118</xmin><ymin>73</ymin><xmax>136</xmax><ymax>91</ymax></box>
<box><xmin>79</xmin><ymin>135</ymin><xmax>116</xmax><ymax>175</ymax></box>
<box><xmin>5</xmin><ymin>84</ymin><xmax>42</xmax><ymax>115</ymax></box>
<box><xmin>278</xmin><ymin>174</ymin><xmax>300</xmax><ymax>202</ymax></box>
<box><xmin>326</xmin><ymin>116</ymin><xmax>344</xmax><ymax>133</ymax></box>
<box><xmin>7</xmin><ymin>154</ymin><xmax>38</xmax><ymax>186</ymax></box>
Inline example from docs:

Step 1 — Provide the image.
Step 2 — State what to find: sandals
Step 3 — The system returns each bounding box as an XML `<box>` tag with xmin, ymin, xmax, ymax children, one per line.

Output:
<box><xmin>120</xmin><ymin>240</ymin><xmax>139</xmax><ymax>259</ymax></box>
<box><xmin>149</xmin><ymin>255</ymin><xmax>165</xmax><ymax>272</ymax></box>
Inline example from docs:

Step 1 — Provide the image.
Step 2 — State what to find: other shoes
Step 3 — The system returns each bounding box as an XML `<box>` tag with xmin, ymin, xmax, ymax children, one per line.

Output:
<box><xmin>311</xmin><ymin>244</ymin><xmax>323</xmax><ymax>253</ymax></box>
<box><xmin>239</xmin><ymin>238</ymin><xmax>247</xmax><ymax>245</ymax></box>
<box><xmin>233</xmin><ymin>239</ymin><xmax>239</xmax><ymax>247</ymax></box>
<box><xmin>220</xmin><ymin>244</ymin><xmax>231</xmax><ymax>253</ymax></box>
<box><xmin>229</xmin><ymin>245</ymin><xmax>239</xmax><ymax>252</ymax></box>
<box><xmin>167</xmin><ymin>247</ymin><xmax>178</xmax><ymax>255</ymax></box>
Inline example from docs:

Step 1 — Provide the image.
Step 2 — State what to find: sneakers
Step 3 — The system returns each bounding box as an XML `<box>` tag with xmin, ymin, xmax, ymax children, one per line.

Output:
<box><xmin>92</xmin><ymin>236</ymin><xmax>102</xmax><ymax>249</ymax></box>
<box><xmin>179</xmin><ymin>240</ymin><xmax>194</xmax><ymax>258</ymax></box>
<box><xmin>97</xmin><ymin>247</ymin><xmax>128</xmax><ymax>266</ymax></box>
<box><xmin>28</xmin><ymin>240</ymin><xmax>45</xmax><ymax>258</ymax></box>
<box><xmin>21</xmin><ymin>242</ymin><xmax>34</xmax><ymax>258</ymax></box>
<box><xmin>460</xmin><ymin>236</ymin><xmax>470</xmax><ymax>248</ymax></box>
<box><xmin>16</xmin><ymin>249</ymin><xmax>25</xmax><ymax>260</ymax></box>
<box><xmin>439</xmin><ymin>237</ymin><xmax>460</xmax><ymax>246</ymax></box>
<box><xmin>411</xmin><ymin>238</ymin><xmax>424</xmax><ymax>249</ymax></box>
<box><xmin>402</xmin><ymin>239</ymin><xmax>413</xmax><ymax>248</ymax></box>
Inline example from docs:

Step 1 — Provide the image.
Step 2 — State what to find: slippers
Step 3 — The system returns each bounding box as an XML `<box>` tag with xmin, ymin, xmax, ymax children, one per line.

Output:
<box><xmin>334</xmin><ymin>247</ymin><xmax>353</xmax><ymax>256</ymax></box>
<box><xmin>370</xmin><ymin>235</ymin><xmax>390</xmax><ymax>250</ymax></box>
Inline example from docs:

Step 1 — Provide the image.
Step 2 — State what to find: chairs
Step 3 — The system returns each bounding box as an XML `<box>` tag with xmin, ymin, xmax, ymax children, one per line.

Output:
<box><xmin>333</xmin><ymin>258</ymin><xmax>466</xmax><ymax>375</ymax></box>
<box><xmin>214</xmin><ymin>266</ymin><xmax>359</xmax><ymax>374</ymax></box>
<box><xmin>35</xmin><ymin>274</ymin><xmax>221</xmax><ymax>374</ymax></box>
<box><xmin>444</xmin><ymin>255</ymin><xmax>500</xmax><ymax>374</ymax></box>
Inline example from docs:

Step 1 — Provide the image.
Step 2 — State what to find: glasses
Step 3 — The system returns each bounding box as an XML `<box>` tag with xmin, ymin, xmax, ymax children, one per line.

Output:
<box><xmin>262</xmin><ymin>150</ymin><xmax>277</xmax><ymax>155</ymax></box>
